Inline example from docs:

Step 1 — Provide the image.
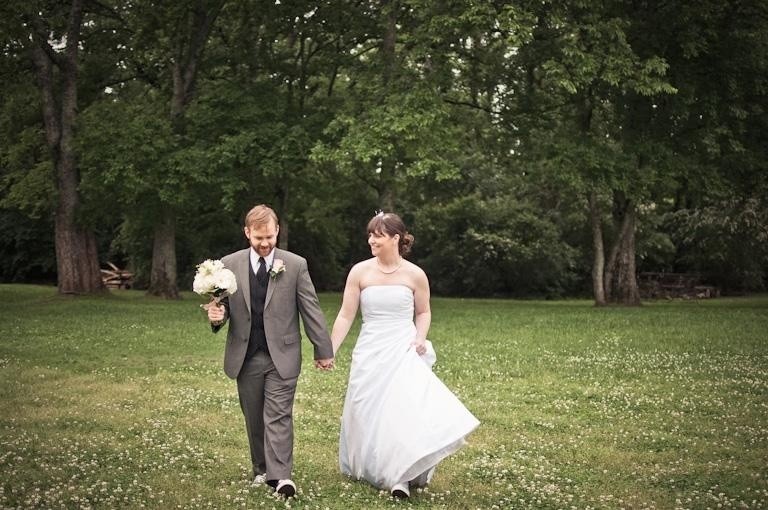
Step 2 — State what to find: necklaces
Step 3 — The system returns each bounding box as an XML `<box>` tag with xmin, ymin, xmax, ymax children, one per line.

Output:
<box><xmin>376</xmin><ymin>255</ymin><xmax>403</xmax><ymax>275</ymax></box>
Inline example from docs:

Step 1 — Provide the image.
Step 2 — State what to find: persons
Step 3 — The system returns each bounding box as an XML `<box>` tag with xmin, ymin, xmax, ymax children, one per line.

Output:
<box><xmin>315</xmin><ymin>209</ymin><xmax>482</xmax><ymax>500</ymax></box>
<box><xmin>206</xmin><ymin>205</ymin><xmax>335</xmax><ymax>497</ymax></box>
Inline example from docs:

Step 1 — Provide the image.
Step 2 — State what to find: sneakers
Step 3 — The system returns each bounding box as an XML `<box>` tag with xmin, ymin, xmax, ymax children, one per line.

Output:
<box><xmin>249</xmin><ymin>471</ymin><xmax>297</xmax><ymax>497</ymax></box>
<box><xmin>391</xmin><ymin>481</ymin><xmax>411</xmax><ymax>500</ymax></box>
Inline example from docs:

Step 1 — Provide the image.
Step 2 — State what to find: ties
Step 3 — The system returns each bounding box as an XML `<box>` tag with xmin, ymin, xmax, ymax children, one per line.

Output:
<box><xmin>256</xmin><ymin>257</ymin><xmax>269</xmax><ymax>288</ymax></box>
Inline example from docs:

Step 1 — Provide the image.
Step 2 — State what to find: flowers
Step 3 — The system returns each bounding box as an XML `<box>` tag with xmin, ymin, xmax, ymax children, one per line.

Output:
<box><xmin>190</xmin><ymin>257</ymin><xmax>238</xmax><ymax>326</ymax></box>
<box><xmin>268</xmin><ymin>258</ymin><xmax>286</xmax><ymax>280</ymax></box>
